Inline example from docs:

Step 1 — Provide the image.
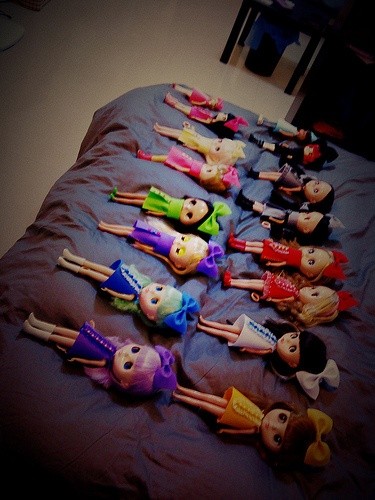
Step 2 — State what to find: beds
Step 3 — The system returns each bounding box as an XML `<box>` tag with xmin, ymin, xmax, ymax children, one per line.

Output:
<box><xmin>0</xmin><ymin>85</ymin><xmax>375</xmax><ymax>500</ymax></box>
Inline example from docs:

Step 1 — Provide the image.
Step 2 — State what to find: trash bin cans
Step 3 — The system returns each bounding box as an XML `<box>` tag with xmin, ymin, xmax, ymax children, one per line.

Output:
<box><xmin>244</xmin><ymin>16</ymin><xmax>301</xmax><ymax>78</ymax></box>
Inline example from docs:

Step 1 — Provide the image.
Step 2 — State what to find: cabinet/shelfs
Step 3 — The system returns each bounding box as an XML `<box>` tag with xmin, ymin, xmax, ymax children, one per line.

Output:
<box><xmin>284</xmin><ymin>0</ymin><xmax>375</xmax><ymax>160</ymax></box>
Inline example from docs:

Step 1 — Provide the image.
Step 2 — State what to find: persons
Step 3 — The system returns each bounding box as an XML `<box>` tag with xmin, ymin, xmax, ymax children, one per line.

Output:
<box><xmin>23</xmin><ymin>82</ymin><xmax>359</xmax><ymax>407</ymax></box>
<box><xmin>172</xmin><ymin>381</ymin><xmax>332</xmax><ymax>477</ymax></box>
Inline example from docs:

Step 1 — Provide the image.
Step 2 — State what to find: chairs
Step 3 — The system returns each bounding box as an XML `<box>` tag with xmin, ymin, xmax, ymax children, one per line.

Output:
<box><xmin>220</xmin><ymin>0</ymin><xmax>330</xmax><ymax>94</ymax></box>
